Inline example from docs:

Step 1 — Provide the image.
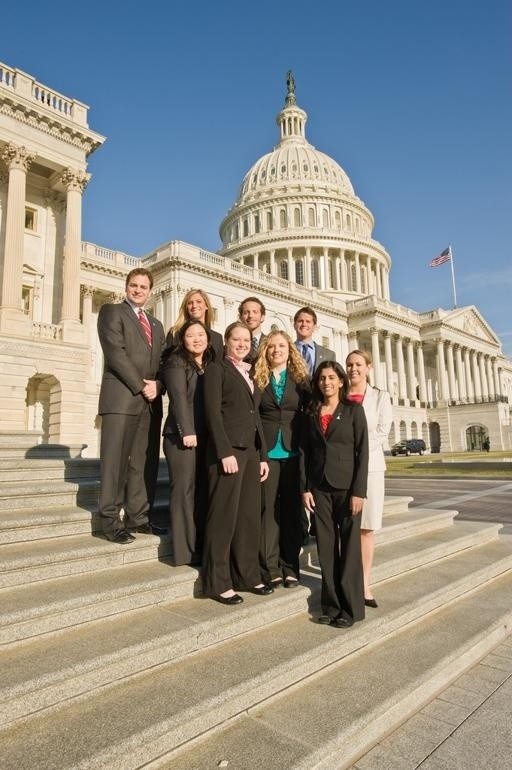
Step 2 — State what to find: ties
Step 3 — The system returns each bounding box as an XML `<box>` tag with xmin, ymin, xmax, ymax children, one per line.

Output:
<box><xmin>139</xmin><ymin>308</ymin><xmax>152</xmax><ymax>347</ymax></box>
<box><xmin>303</xmin><ymin>345</ymin><xmax>312</xmax><ymax>374</ymax></box>
<box><xmin>252</xmin><ymin>338</ymin><xmax>258</xmax><ymax>353</ymax></box>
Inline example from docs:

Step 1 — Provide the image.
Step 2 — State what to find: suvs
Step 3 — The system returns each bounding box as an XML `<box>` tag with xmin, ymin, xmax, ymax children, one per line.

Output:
<box><xmin>390</xmin><ymin>439</ymin><xmax>425</xmax><ymax>455</ymax></box>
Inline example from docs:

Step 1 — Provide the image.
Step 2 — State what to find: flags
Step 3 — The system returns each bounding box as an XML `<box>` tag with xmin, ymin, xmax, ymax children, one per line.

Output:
<box><xmin>431</xmin><ymin>247</ymin><xmax>451</xmax><ymax>267</ymax></box>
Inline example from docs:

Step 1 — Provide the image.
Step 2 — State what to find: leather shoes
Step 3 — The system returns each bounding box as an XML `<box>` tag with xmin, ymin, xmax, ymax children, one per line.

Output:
<box><xmin>250</xmin><ymin>577</ymin><xmax>299</xmax><ymax>595</ymax></box>
<box><xmin>212</xmin><ymin>594</ymin><xmax>243</xmax><ymax>604</ymax></box>
<box><xmin>126</xmin><ymin>522</ymin><xmax>167</xmax><ymax>534</ymax></box>
<box><xmin>364</xmin><ymin>599</ymin><xmax>377</xmax><ymax>607</ymax></box>
<box><xmin>100</xmin><ymin>528</ymin><xmax>136</xmax><ymax>543</ymax></box>
<box><xmin>319</xmin><ymin>615</ymin><xmax>355</xmax><ymax>628</ymax></box>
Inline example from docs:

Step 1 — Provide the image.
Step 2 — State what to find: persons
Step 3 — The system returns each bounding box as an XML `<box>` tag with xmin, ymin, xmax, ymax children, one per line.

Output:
<box><xmin>238</xmin><ymin>297</ymin><xmax>267</xmax><ymax>365</ymax></box>
<box><xmin>252</xmin><ymin>331</ymin><xmax>314</xmax><ymax>589</ymax></box>
<box><xmin>296</xmin><ymin>361</ymin><xmax>369</xmax><ymax>628</ymax></box>
<box><xmin>166</xmin><ymin>289</ymin><xmax>224</xmax><ymax>363</ymax></box>
<box><xmin>201</xmin><ymin>322</ymin><xmax>274</xmax><ymax>605</ymax></box>
<box><xmin>98</xmin><ymin>268</ymin><xmax>169</xmax><ymax>544</ymax></box>
<box><xmin>162</xmin><ymin>320</ymin><xmax>217</xmax><ymax>566</ymax></box>
<box><xmin>346</xmin><ymin>350</ymin><xmax>392</xmax><ymax>608</ymax></box>
<box><xmin>291</xmin><ymin>307</ymin><xmax>337</xmax><ymax>545</ymax></box>
